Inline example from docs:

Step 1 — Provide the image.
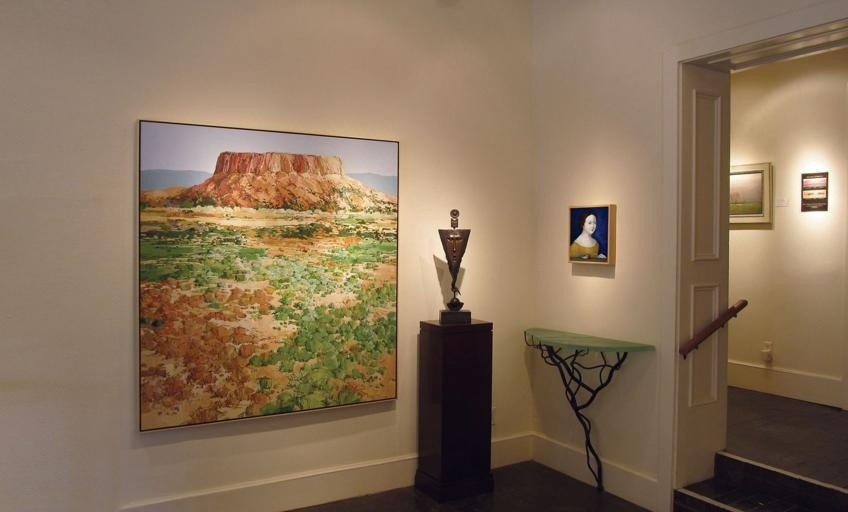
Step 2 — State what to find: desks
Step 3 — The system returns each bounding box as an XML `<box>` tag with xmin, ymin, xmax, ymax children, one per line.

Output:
<box><xmin>522</xmin><ymin>326</ymin><xmax>656</xmax><ymax>491</ymax></box>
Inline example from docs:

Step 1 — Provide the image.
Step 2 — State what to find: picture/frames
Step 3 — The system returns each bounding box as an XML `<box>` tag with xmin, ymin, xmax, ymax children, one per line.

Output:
<box><xmin>729</xmin><ymin>162</ymin><xmax>774</xmax><ymax>224</ymax></box>
<box><xmin>567</xmin><ymin>204</ymin><xmax>617</xmax><ymax>266</ymax></box>
<box><xmin>137</xmin><ymin>118</ymin><xmax>400</xmax><ymax>434</ymax></box>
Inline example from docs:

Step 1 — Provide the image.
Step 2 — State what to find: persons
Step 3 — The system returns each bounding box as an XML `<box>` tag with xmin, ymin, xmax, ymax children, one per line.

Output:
<box><xmin>569</xmin><ymin>208</ymin><xmax>607</xmax><ymax>259</ymax></box>
